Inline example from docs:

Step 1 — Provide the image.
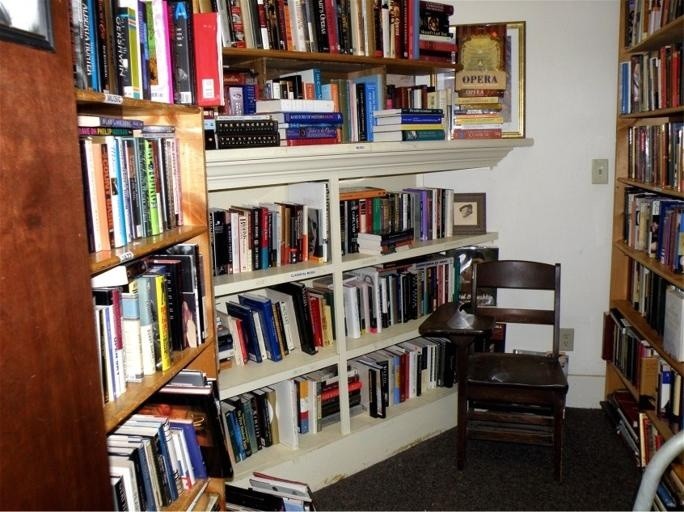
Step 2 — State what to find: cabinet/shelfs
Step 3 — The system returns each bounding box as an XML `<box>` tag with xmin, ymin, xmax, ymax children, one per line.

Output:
<box><xmin>604</xmin><ymin>0</ymin><xmax>684</xmax><ymax>510</ymax></box>
<box><xmin>204</xmin><ymin>137</ymin><xmax>536</xmax><ymax>493</ymax></box>
<box><xmin>1</xmin><ymin>35</ymin><xmax>220</xmax><ymax>511</ymax></box>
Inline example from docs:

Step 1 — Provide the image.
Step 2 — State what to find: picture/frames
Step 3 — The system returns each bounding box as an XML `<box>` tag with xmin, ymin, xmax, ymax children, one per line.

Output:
<box><xmin>452</xmin><ymin>191</ymin><xmax>487</xmax><ymax>235</ymax></box>
<box><xmin>428</xmin><ymin>20</ymin><xmax>527</xmax><ymax>140</ymax></box>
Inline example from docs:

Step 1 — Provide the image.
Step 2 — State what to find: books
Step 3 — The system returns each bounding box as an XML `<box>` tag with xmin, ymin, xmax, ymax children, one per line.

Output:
<box><xmin>338</xmin><ymin>187</ymin><xmax>499</xmax><ymax>339</ymax></box>
<box><xmin>106</xmin><ymin>366</ymin><xmax>317</xmax><ymax>512</ymax></box>
<box><xmin>78</xmin><ymin>116</ymin><xmax>207</xmax><ymax>407</ymax></box>
<box><xmin>622</xmin><ymin>116</ymin><xmax>683</xmax><ymax>275</ymax></box>
<box><xmin>304</xmin><ymin>337</ymin><xmax>460</xmax><ymax>434</ymax></box>
<box><xmin>206</xmin><ymin>183</ymin><xmax>338</xmax><ymax>369</ymax></box>
<box><xmin>601</xmin><ymin>258</ymin><xmax>684</xmax><ymax>510</ymax></box>
<box><xmin>621</xmin><ymin>1</ymin><xmax>683</xmax><ymax>114</ymax></box>
<box><xmin>73</xmin><ymin>0</ymin><xmax>525</xmax><ymax>150</ymax></box>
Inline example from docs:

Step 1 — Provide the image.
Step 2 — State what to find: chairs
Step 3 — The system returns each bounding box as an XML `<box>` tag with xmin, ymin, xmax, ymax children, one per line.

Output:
<box><xmin>455</xmin><ymin>256</ymin><xmax>571</xmax><ymax>483</ymax></box>
